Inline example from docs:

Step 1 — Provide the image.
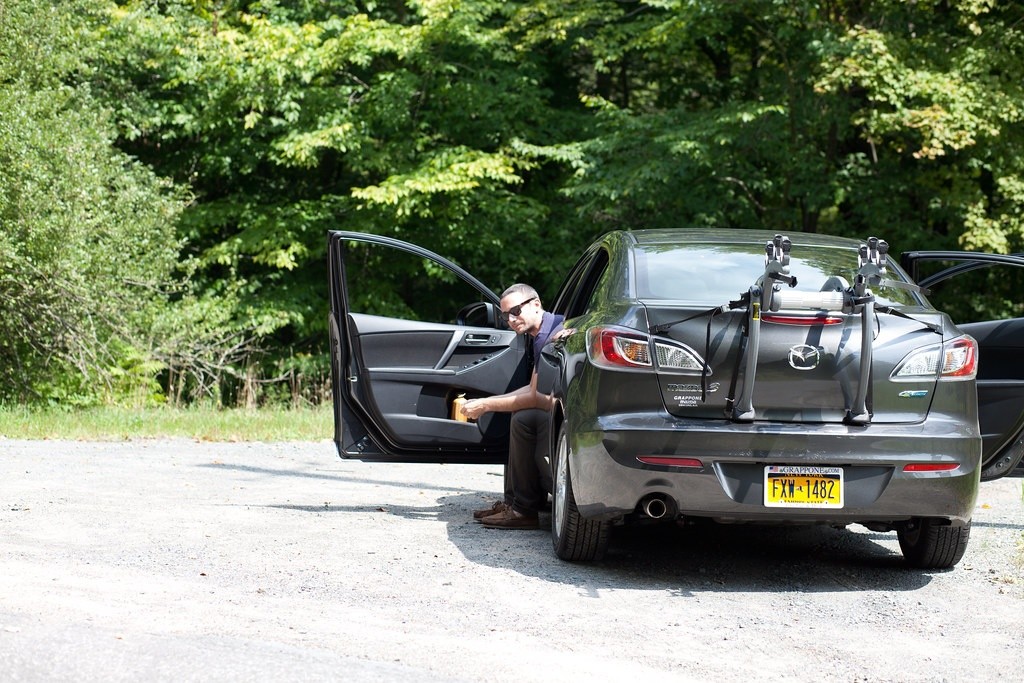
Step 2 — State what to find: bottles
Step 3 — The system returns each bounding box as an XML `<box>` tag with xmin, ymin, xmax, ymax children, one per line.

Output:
<box><xmin>452</xmin><ymin>395</ymin><xmax>468</xmax><ymax>423</ymax></box>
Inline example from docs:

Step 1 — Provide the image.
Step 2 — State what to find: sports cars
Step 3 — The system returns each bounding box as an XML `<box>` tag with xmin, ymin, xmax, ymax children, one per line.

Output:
<box><xmin>322</xmin><ymin>224</ymin><xmax>1024</xmax><ymax>570</ymax></box>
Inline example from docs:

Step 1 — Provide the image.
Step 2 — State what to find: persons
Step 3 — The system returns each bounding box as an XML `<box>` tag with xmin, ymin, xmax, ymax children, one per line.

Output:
<box><xmin>460</xmin><ymin>284</ymin><xmax>566</xmax><ymax>530</ymax></box>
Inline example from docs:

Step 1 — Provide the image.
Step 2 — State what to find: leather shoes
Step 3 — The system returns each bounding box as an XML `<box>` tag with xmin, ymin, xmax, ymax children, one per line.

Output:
<box><xmin>473</xmin><ymin>500</ymin><xmax>508</xmax><ymax>521</ymax></box>
<box><xmin>482</xmin><ymin>506</ymin><xmax>539</xmax><ymax>529</ymax></box>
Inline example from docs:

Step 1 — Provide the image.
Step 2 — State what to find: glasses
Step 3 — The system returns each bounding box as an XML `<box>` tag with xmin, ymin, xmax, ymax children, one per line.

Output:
<box><xmin>502</xmin><ymin>298</ymin><xmax>537</xmax><ymax>322</ymax></box>
